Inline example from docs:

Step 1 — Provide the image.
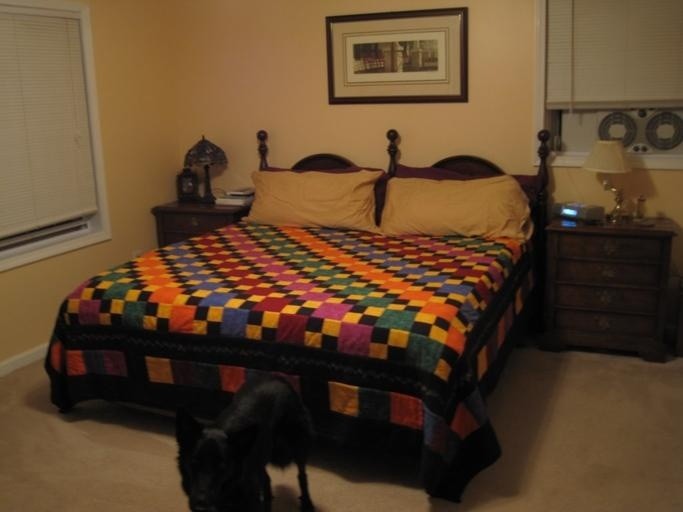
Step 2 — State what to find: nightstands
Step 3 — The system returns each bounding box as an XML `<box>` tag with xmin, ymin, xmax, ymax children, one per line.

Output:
<box><xmin>150</xmin><ymin>195</ymin><xmax>249</xmax><ymax>243</ymax></box>
<box><xmin>544</xmin><ymin>212</ymin><xmax>678</xmax><ymax>364</ymax></box>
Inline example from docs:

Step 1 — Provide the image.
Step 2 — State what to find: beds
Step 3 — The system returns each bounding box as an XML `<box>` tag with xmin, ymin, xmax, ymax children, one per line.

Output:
<box><xmin>43</xmin><ymin>128</ymin><xmax>547</xmax><ymax>511</ymax></box>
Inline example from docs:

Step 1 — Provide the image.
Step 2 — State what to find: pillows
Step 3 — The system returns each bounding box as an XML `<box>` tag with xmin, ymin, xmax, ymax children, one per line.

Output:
<box><xmin>244</xmin><ymin>168</ymin><xmax>382</xmax><ymax>233</ymax></box>
<box><xmin>380</xmin><ymin>175</ymin><xmax>534</xmax><ymax>241</ymax></box>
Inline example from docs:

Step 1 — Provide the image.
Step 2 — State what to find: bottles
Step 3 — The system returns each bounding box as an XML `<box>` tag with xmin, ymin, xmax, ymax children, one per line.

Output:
<box><xmin>176</xmin><ymin>167</ymin><xmax>196</xmax><ymax>200</ymax></box>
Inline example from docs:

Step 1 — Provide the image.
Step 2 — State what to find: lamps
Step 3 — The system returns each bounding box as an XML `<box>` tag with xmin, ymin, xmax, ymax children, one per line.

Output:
<box><xmin>184</xmin><ymin>134</ymin><xmax>228</xmax><ymax>203</ymax></box>
<box><xmin>584</xmin><ymin>140</ymin><xmax>627</xmax><ymax>220</ymax></box>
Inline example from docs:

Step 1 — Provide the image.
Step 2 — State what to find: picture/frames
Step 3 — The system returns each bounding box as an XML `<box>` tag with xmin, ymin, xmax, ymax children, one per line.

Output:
<box><xmin>325</xmin><ymin>6</ymin><xmax>470</xmax><ymax>104</ymax></box>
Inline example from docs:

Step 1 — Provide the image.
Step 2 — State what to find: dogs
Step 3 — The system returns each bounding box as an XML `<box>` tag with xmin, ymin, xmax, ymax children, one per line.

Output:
<box><xmin>175</xmin><ymin>375</ymin><xmax>315</xmax><ymax>512</ymax></box>
